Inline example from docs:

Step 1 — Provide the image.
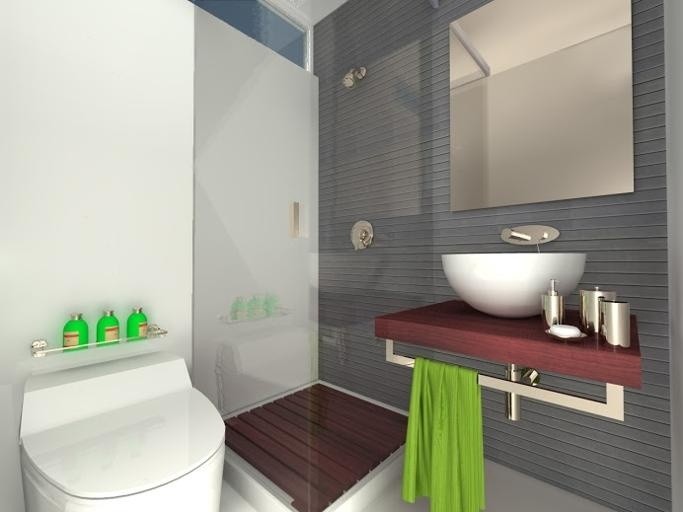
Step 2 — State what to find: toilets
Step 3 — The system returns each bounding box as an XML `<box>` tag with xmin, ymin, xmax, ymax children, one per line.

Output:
<box><xmin>20</xmin><ymin>351</ymin><xmax>226</xmax><ymax>511</ymax></box>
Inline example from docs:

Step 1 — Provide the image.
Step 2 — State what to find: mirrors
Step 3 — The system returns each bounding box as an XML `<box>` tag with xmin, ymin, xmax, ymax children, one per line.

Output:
<box><xmin>448</xmin><ymin>1</ymin><xmax>635</xmax><ymax>212</ymax></box>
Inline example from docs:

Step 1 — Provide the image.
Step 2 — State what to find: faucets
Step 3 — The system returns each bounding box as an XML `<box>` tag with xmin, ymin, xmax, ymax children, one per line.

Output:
<box><xmin>501</xmin><ymin>228</ymin><xmax>530</xmax><ymax>244</ymax></box>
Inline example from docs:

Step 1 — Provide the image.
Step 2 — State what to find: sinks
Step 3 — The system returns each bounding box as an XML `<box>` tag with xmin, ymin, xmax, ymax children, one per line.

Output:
<box><xmin>441</xmin><ymin>253</ymin><xmax>588</xmax><ymax>318</ymax></box>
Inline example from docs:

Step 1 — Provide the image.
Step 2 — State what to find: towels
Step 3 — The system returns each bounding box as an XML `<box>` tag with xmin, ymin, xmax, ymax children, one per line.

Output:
<box><xmin>402</xmin><ymin>357</ymin><xmax>485</xmax><ymax>512</ymax></box>
